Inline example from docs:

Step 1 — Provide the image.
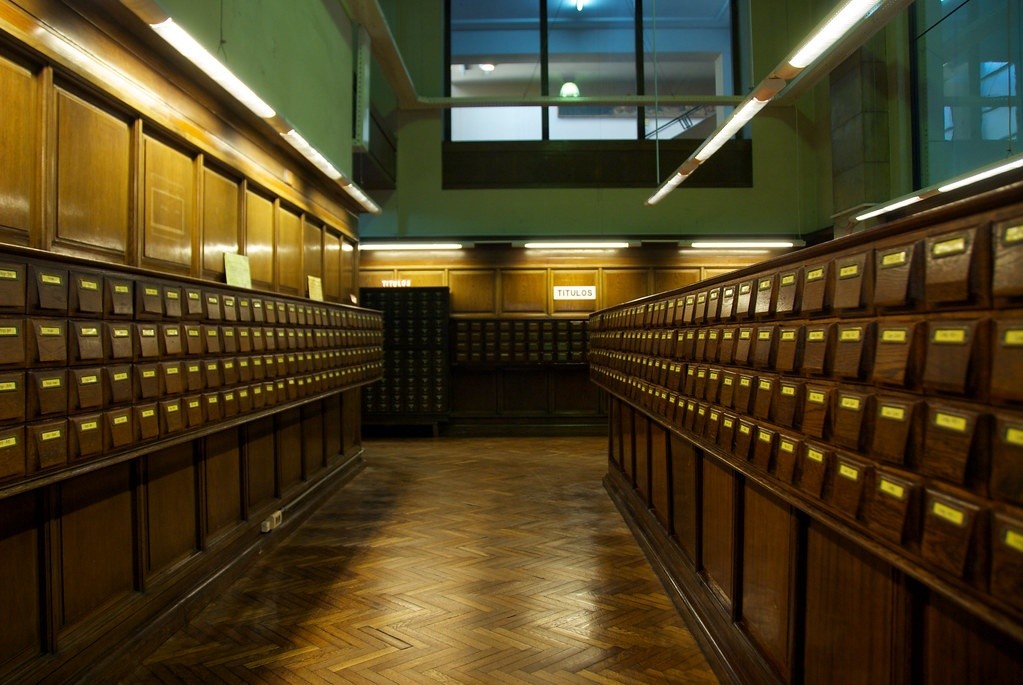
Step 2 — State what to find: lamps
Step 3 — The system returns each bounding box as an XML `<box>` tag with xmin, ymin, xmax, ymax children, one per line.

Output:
<box><xmin>851</xmin><ymin>1</ymin><xmax>1023</xmax><ymax>222</ymax></box>
<box><xmin>647</xmin><ymin>0</ymin><xmax>888</xmax><ymax>205</ymax></box>
<box><xmin>118</xmin><ymin>0</ymin><xmax>382</xmax><ymax>216</ymax></box>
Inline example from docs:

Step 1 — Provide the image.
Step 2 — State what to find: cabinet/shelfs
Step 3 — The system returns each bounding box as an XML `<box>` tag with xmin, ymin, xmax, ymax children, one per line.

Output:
<box><xmin>451</xmin><ymin>315</ymin><xmax>587</xmax><ymax>369</ymax></box>
<box><xmin>587</xmin><ymin>180</ymin><xmax>1023</xmax><ymax>642</ymax></box>
<box><xmin>358</xmin><ymin>286</ymin><xmax>451</xmax><ymax>440</ymax></box>
<box><xmin>0</xmin><ymin>242</ymin><xmax>385</xmax><ymax>500</ymax></box>
